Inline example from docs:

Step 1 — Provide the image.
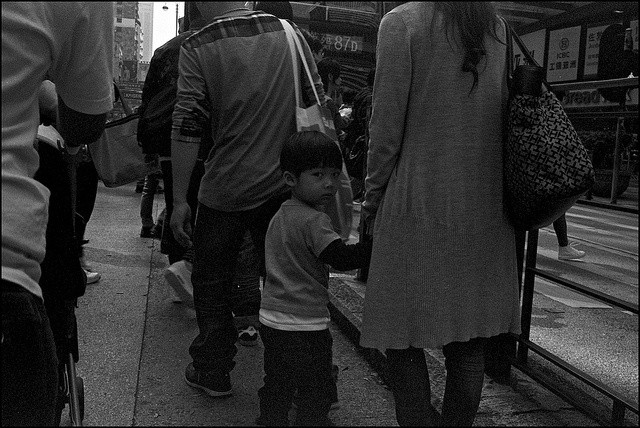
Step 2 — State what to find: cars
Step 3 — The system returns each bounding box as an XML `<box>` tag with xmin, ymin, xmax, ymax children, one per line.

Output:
<box><xmin>592</xmin><ymin>170</ymin><xmax>630</xmax><ymax>196</ymax></box>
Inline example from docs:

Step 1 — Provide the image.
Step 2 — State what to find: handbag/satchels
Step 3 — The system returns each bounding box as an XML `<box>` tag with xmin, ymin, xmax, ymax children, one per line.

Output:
<box><xmin>90</xmin><ymin>81</ymin><xmax>162</xmax><ymax>188</ymax></box>
<box><xmin>279</xmin><ymin>19</ymin><xmax>354</xmax><ymax>241</ymax></box>
<box><xmin>499</xmin><ymin>15</ymin><xmax>595</xmax><ymax>231</ymax></box>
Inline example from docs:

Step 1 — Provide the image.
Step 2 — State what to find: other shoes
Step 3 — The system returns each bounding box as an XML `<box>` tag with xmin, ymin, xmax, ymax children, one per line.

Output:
<box><xmin>141</xmin><ymin>230</ymin><xmax>156</xmax><ymax>238</ymax></box>
<box><xmin>237</xmin><ymin>324</ymin><xmax>257</xmax><ymax>345</ymax></box>
<box><xmin>185</xmin><ymin>362</ymin><xmax>232</xmax><ymax>396</ymax></box>
<box><xmin>136</xmin><ymin>180</ymin><xmax>159</xmax><ymax>193</ymax></box>
<box><xmin>164</xmin><ymin>259</ymin><xmax>194</xmax><ymax>309</ymax></box>
<box><xmin>82</xmin><ymin>267</ymin><xmax>102</xmax><ymax>284</ymax></box>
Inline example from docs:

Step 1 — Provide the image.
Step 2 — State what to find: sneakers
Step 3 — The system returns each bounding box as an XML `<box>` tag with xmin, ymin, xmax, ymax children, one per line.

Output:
<box><xmin>558</xmin><ymin>241</ymin><xmax>586</xmax><ymax>260</ymax></box>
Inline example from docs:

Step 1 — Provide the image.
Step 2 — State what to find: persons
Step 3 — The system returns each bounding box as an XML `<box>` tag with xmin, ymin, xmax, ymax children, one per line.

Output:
<box><xmin>2</xmin><ymin>2</ymin><xmax>523</xmax><ymax>428</ymax></box>
<box><xmin>257</xmin><ymin>128</ymin><xmax>372</xmax><ymax>427</ymax></box>
<box><xmin>553</xmin><ymin>212</ymin><xmax>585</xmax><ymax>260</ymax></box>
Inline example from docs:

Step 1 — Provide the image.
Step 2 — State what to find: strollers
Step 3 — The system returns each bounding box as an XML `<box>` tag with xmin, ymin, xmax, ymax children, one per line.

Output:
<box><xmin>32</xmin><ymin>134</ymin><xmax>90</xmax><ymax>428</ymax></box>
